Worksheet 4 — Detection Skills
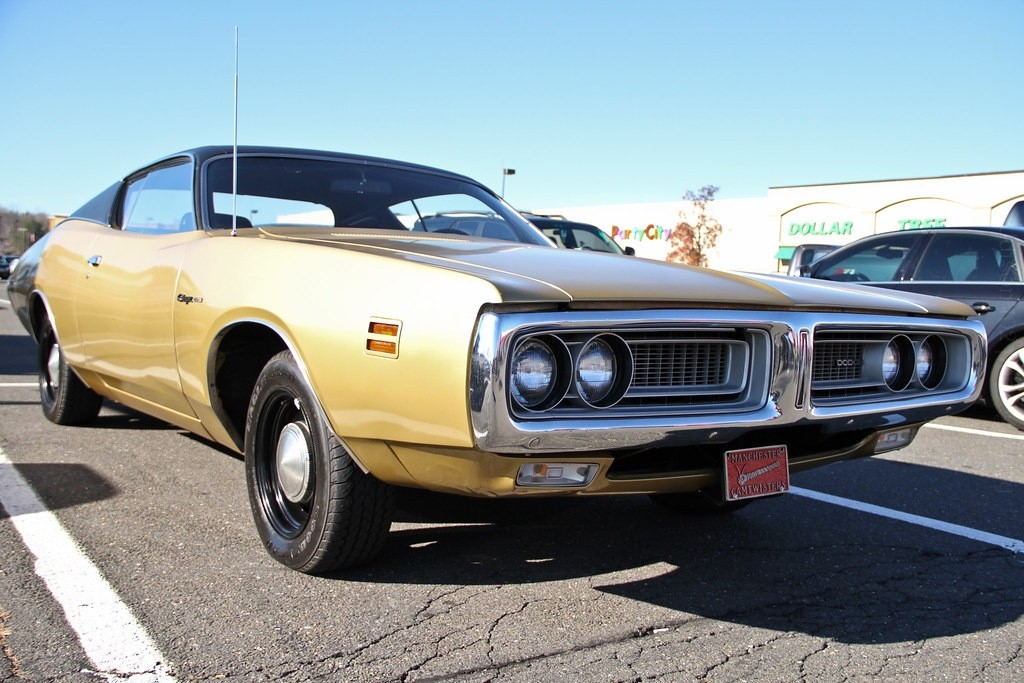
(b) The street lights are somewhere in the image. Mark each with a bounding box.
[502,168,515,200]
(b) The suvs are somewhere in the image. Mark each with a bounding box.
[411,211,636,256]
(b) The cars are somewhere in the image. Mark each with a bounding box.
[7,145,988,578]
[0,261,11,280]
[0,255,20,265]
[800,225,1023,432]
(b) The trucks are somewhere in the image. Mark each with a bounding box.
[788,244,1000,281]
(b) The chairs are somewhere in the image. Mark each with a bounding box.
[178,213,252,232]
[965,250,1002,280]
[916,253,953,281]
